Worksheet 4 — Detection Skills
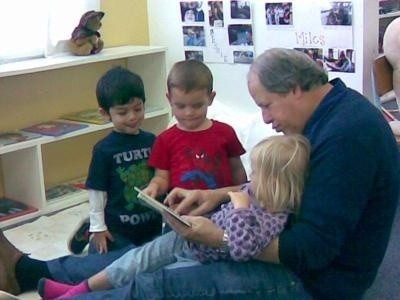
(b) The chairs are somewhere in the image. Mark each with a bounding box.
[370,52,400,143]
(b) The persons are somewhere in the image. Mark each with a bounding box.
[139,59,247,235]
[67,65,159,256]
[0,47,398,299]
[180,1,354,72]
[39,133,312,299]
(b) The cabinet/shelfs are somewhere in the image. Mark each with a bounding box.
[1,41,173,237]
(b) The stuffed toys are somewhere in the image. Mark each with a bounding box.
[69,10,104,55]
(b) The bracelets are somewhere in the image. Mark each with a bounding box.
[217,230,228,253]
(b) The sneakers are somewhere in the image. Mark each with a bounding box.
[0,228,31,295]
[67,216,93,254]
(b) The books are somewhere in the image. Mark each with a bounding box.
[0,107,112,222]
[133,185,191,228]
